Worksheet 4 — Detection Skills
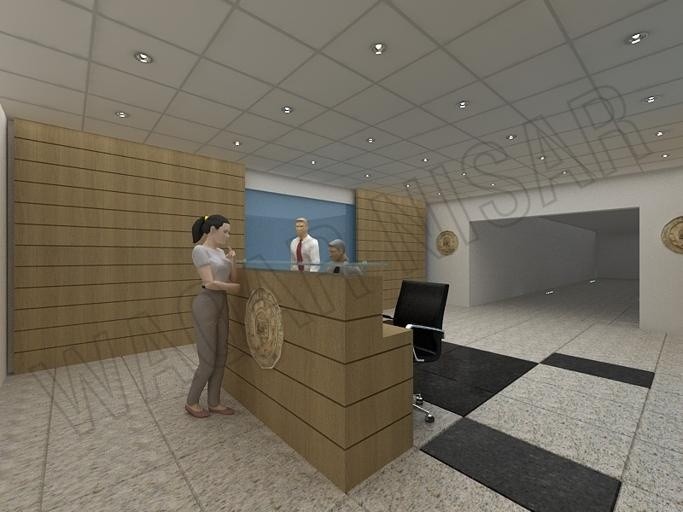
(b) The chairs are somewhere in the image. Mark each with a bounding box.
[382,280,449,421]
